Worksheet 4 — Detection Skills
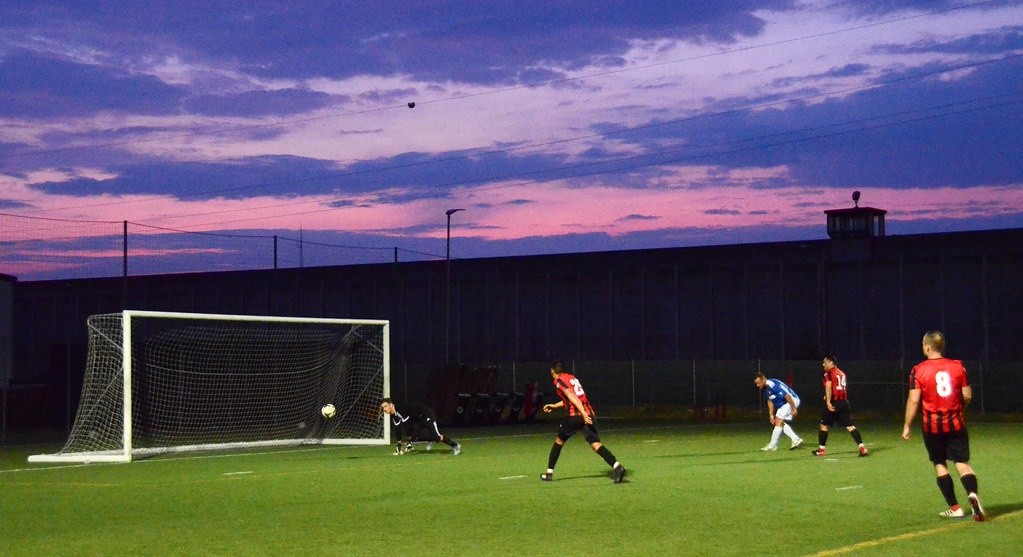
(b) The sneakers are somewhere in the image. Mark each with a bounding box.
[859,447,868,456]
[790,438,803,450]
[967,492,985,522]
[812,448,826,455]
[938,506,964,517]
[540,473,553,481]
[614,466,626,482]
[761,444,777,452]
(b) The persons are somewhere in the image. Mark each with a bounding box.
[811,355,869,457]
[381,397,462,456]
[901,330,986,522]
[754,373,804,452]
[540,360,627,485]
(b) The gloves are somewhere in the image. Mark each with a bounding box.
[405,443,413,452]
[393,444,404,456]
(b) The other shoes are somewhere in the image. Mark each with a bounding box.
[453,443,461,455]
[426,441,433,451]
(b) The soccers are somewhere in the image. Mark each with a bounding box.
[321,404,336,418]
